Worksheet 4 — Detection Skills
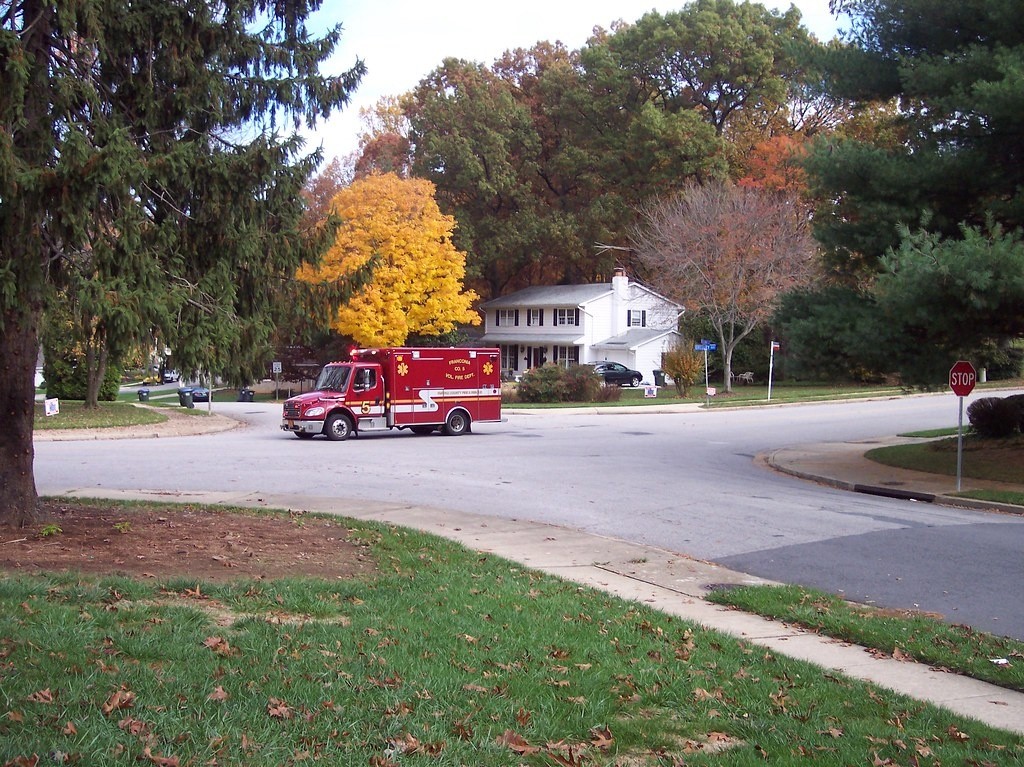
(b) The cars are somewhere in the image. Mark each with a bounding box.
[142,372,167,385]
[162,370,179,383]
[176,386,214,402]
[582,361,643,389]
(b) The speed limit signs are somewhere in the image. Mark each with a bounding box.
[273,362,282,373]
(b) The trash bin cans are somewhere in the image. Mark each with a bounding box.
[177,388,194,407]
[979,367,987,383]
[137,389,150,402]
[238,389,256,402]
[652,369,667,387]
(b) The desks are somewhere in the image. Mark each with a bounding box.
[735,376,742,381]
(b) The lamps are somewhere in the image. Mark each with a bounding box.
[520,345,524,352]
[543,346,547,353]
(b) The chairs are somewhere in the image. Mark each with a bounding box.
[730,372,754,384]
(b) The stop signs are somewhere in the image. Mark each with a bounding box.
[948,361,975,398]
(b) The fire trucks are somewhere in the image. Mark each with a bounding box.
[279,346,507,442]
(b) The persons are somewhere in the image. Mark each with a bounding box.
[355,371,375,391]
